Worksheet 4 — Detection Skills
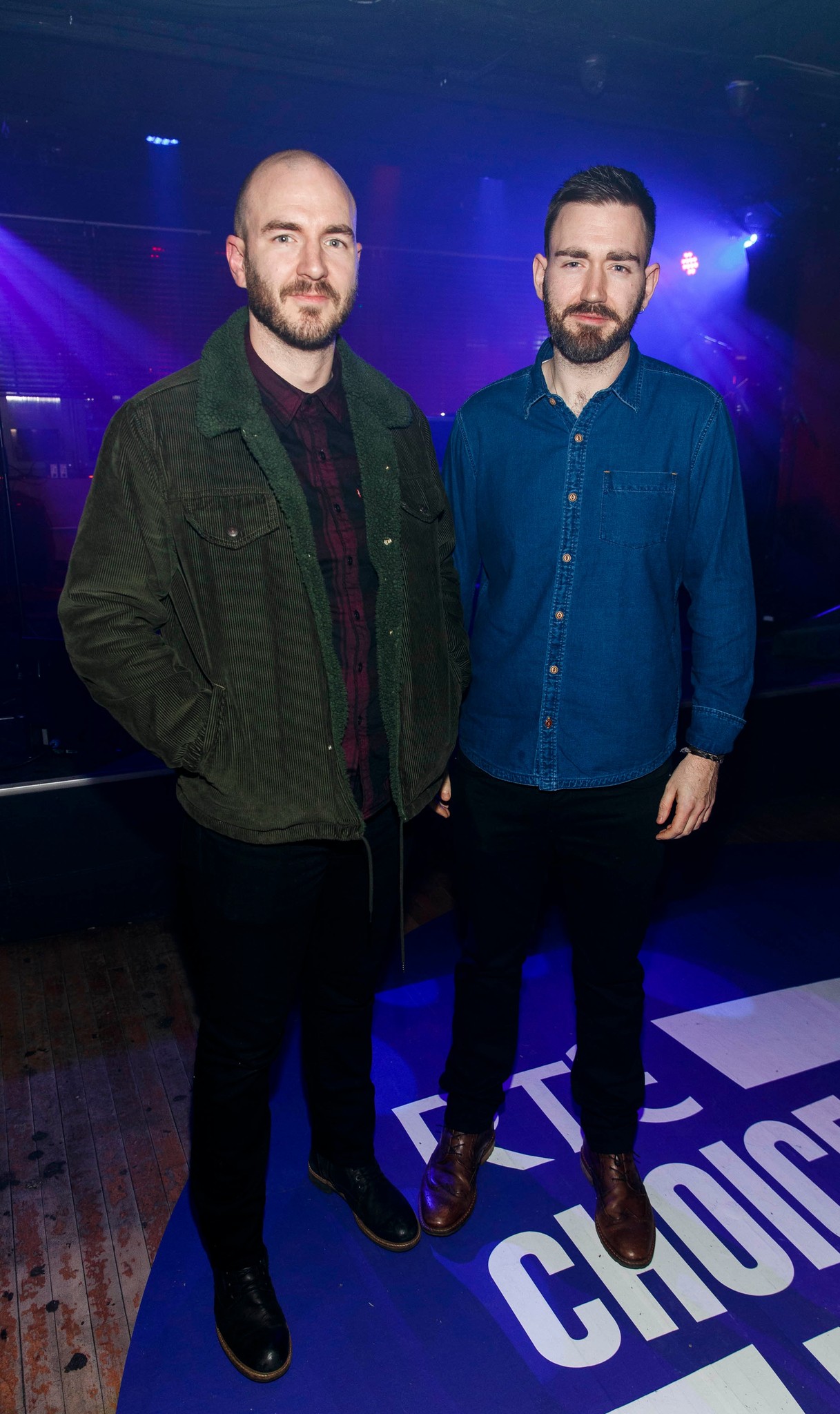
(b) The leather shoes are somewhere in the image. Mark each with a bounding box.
[419,1125,496,1235]
[308,1141,420,1248]
[581,1140,658,1270]
[213,1249,292,1378]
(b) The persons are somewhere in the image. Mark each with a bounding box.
[421,163,755,1269]
[59,149,468,1379]
